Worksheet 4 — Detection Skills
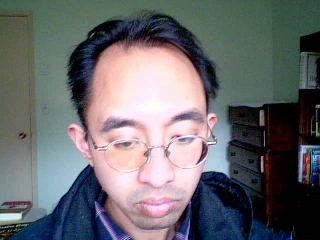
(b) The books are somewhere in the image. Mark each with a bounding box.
[297,145,320,186]
[299,51,320,89]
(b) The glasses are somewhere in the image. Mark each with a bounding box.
[87,124,222,174]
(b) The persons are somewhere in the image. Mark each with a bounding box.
[8,8,294,240]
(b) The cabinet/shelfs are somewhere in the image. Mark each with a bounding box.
[227,102,297,231]
[297,37,320,239]
